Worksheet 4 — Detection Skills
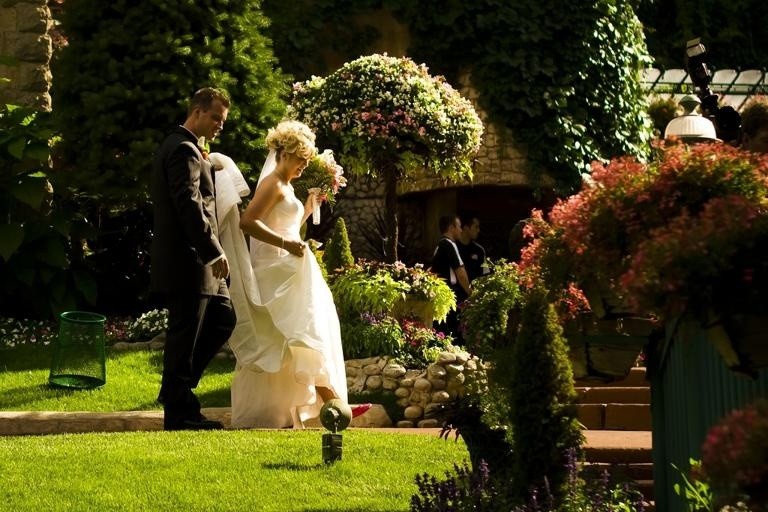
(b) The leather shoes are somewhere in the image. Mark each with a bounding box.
[163,416,225,431]
[351,402,372,419]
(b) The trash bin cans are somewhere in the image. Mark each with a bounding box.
[48,310,108,390]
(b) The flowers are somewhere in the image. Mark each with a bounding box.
[296,150,346,214]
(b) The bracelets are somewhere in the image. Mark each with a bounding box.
[281,236,285,250]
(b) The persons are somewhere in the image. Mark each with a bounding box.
[150,87,230,430]
[739,93,768,154]
[432,210,487,345]
[224,120,371,429]
